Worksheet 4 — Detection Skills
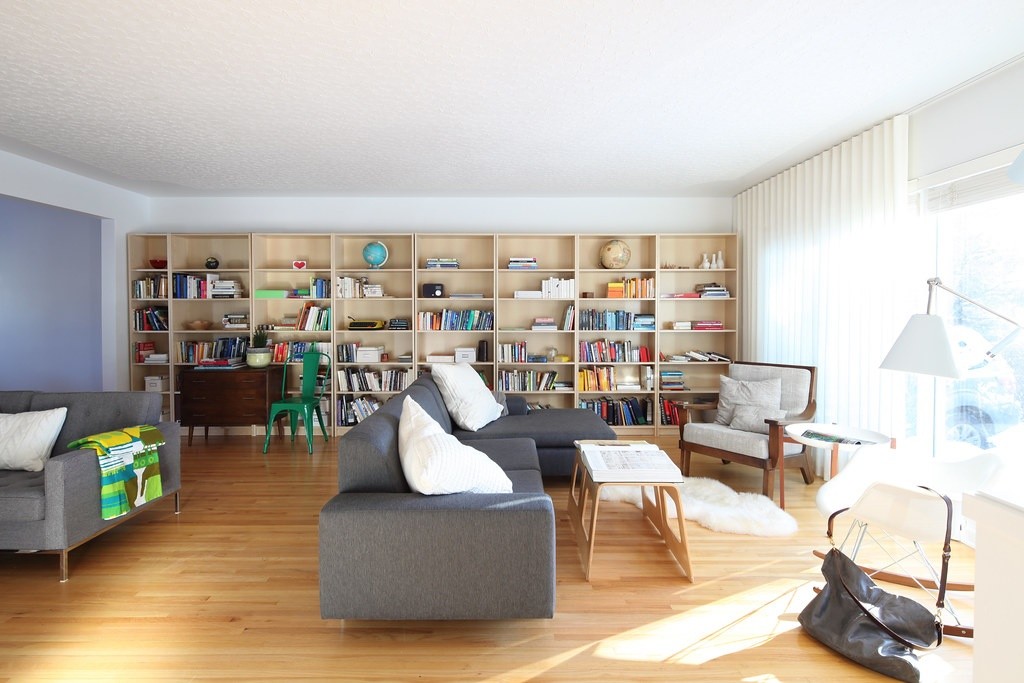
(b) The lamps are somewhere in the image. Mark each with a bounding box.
[877,276,1024,382]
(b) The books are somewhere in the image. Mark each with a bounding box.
[336,276,395,297]
[418,368,431,378]
[337,342,412,426]
[255,272,331,391]
[659,282,731,425]
[132,273,169,363]
[497,257,574,410]
[172,272,250,363]
[418,307,494,330]
[579,275,654,426]
[425,258,460,270]
[477,369,493,391]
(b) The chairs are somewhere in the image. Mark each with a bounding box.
[813,442,975,625]
[262,351,331,456]
[671,360,819,503]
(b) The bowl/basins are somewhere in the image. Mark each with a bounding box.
[187,322,213,330]
[150,259,167,268]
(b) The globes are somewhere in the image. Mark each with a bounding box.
[596,240,633,270]
[363,240,390,271]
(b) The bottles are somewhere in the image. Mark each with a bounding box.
[709,253,717,269]
[699,255,710,269]
[717,250,724,268]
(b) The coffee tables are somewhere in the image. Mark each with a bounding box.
[568,439,695,582]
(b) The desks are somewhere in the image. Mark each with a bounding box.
[785,423,891,480]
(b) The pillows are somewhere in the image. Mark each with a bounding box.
[729,404,789,435]
[713,374,782,426]
[431,364,505,432]
[0,406,68,473]
[397,393,515,496]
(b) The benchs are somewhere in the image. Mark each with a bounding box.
[0,389,183,582]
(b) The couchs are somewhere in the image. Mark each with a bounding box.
[408,372,617,478]
[314,384,555,622]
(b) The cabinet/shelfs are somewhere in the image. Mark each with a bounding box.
[962,469,1024,683]
[577,233,657,437]
[126,233,173,423]
[333,232,415,438]
[251,233,334,436]
[176,365,289,448]
[169,232,253,437]
[495,233,577,408]
[414,232,496,391]
[657,233,739,438]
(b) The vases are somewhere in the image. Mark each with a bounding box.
[710,254,717,269]
[717,250,725,269]
[702,254,710,269]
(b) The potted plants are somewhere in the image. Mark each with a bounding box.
[245,324,273,369]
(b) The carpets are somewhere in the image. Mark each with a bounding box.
[598,475,798,537]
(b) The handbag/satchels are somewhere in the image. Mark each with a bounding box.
[798,484,952,683]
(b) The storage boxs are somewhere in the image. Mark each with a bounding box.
[314,396,328,401]
[292,260,306,269]
[356,345,385,363]
[159,406,171,423]
[305,400,331,414]
[605,282,624,298]
[312,415,330,426]
[381,353,389,362]
[363,284,384,298]
[513,290,542,299]
[426,355,455,363]
[455,347,477,362]
[674,326,692,330]
[583,292,595,298]
[143,374,170,392]
[673,322,692,327]
